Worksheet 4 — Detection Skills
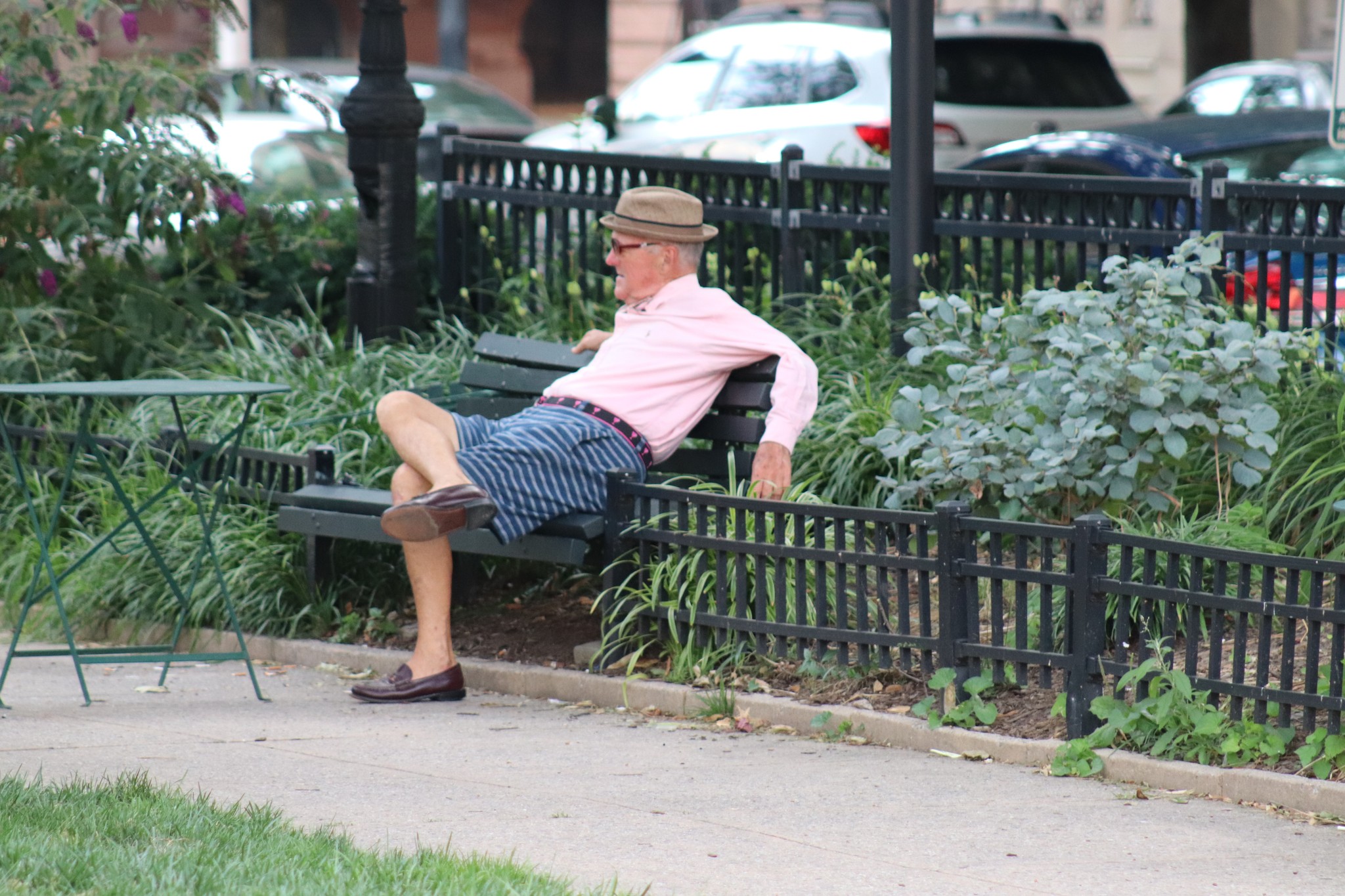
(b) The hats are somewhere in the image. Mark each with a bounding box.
[600,185,718,243]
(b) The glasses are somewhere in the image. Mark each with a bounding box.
[612,238,660,253]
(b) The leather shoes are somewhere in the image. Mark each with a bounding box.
[350,660,468,705]
[380,482,498,544]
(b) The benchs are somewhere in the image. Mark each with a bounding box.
[268,324,785,576]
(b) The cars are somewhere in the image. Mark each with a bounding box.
[941,106,1345,374]
[1158,56,1345,128]
[30,55,537,277]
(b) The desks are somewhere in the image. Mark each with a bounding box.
[0,374,294,709]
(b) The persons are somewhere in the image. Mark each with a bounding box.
[350,185,819,704]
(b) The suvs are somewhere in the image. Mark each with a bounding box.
[494,6,1147,284]
